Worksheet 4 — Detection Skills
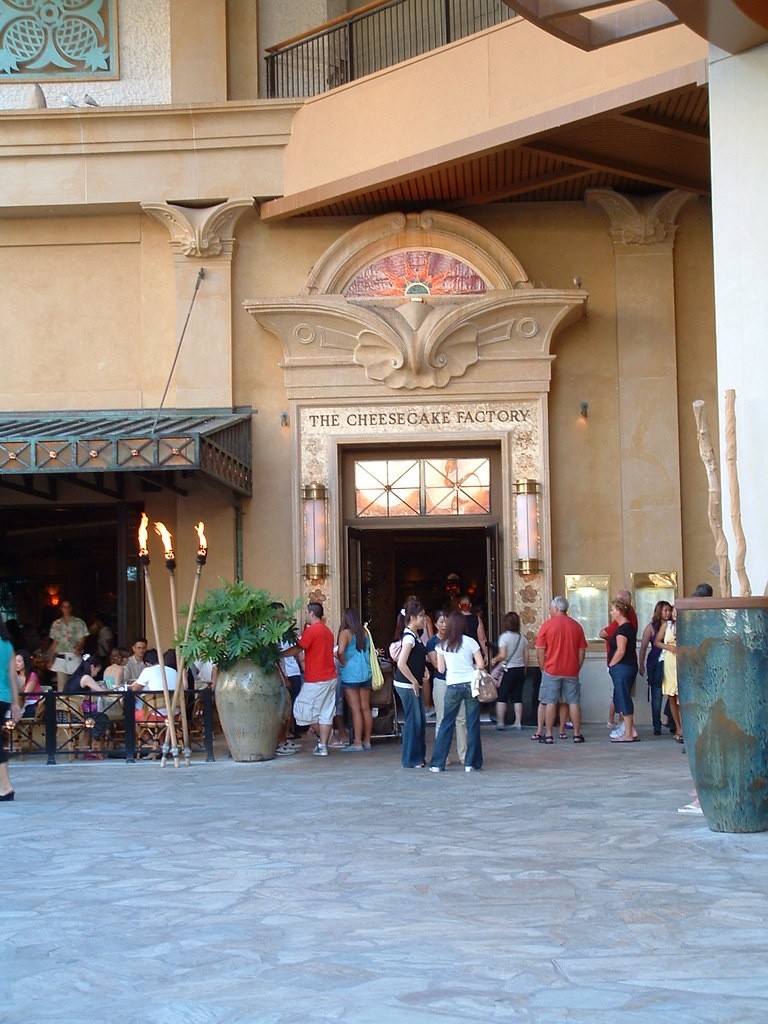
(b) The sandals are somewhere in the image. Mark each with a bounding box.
[559,733,567,739]
[539,735,553,744]
[531,734,545,740]
[573,734,585,742]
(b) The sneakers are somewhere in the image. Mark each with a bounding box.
[275,745,295,755]
[317,729,333,746]
[313,745,328,756]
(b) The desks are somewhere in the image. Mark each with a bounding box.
[97,696,124,721]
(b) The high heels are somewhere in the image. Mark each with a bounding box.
[0,791,15,801]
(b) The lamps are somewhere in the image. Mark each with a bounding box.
[280,411,288,426]
[511,478,543,575]
[300,481,330,581]
[580,402,589,418]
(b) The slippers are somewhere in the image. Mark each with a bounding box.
[612,737,640,743]
[677,804,703,813]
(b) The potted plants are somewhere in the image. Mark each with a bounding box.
[170,577,306,762]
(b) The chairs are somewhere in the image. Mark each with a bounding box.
[105,693,204,761]
[1,695,104,763]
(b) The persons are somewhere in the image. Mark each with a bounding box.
[427,611,468,765]
[258,602,350,755]
[46,600,91,691]
[394,593,489,717]
[429,610,485,773]
[392,601,430,769]
[85,612,218,759]
[279,602,338,756]
[5,618,58,730]
[491,612,529,730]
[531,583,713,744]
[0,611,23,802]
[63,654,111,761]
[337,607,385,753]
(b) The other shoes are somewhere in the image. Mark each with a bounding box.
[464,766,474,773]
[496,723,506,730]
[143,753,162,760]
[429,766,440,773]
[610,722,625,738]
[328,736,349,748]
[362,740,371,751]
[672,733,683,743]
[402,762,425,768]
[507,724,522,730]
[339,744,364,752]
[607,721,617,729]
[284,742,304,752]
[83,753,107,760]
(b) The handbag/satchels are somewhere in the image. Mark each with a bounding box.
[82,700,97,712]
[365,627,385,691]
[491,662,505,688]
[478,667,498,703]
